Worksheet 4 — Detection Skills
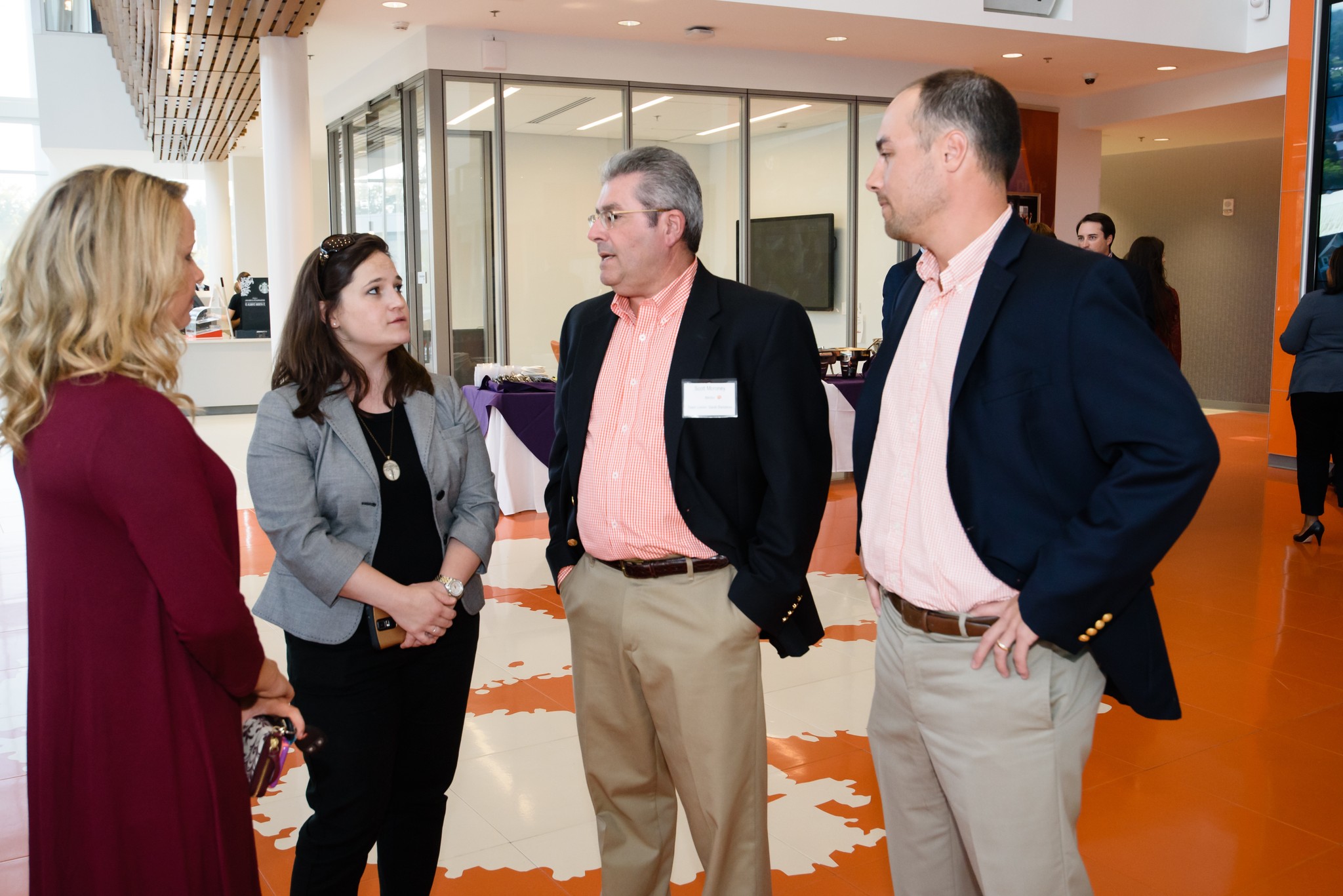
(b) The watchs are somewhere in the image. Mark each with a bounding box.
[434,574,464,599]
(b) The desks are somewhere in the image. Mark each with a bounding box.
[461,385,555,515]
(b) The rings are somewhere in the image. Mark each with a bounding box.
[431,626,438,635]
[996,639,1011,652]
[427,633,434,638]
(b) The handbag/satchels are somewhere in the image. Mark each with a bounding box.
[242,714,298,799]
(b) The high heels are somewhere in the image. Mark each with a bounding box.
[1293,520,1325,547]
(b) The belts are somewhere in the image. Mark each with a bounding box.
[595,553,731,579]
[886,591,999,638]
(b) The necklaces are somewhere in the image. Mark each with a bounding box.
[350,388,401,481]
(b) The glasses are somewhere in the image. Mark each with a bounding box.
[588,207,671,231]
[318,232,361,288]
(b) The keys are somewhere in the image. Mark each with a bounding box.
[272,717,295,741]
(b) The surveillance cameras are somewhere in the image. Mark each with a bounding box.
[1080,72,1099,85]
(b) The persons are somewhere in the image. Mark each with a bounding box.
[1278,244,1343,547]
[1076,212,1120,259]
[245,224,497,896]
[228,271,252,337]
[1122,236,1183,369]
[195,282,209,291]
[1027,222,1058,239]
[0,163,309,896]
[848,66,1227,895]
[545,147,837,896]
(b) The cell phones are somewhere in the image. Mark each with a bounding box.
[365,603,407,651]
